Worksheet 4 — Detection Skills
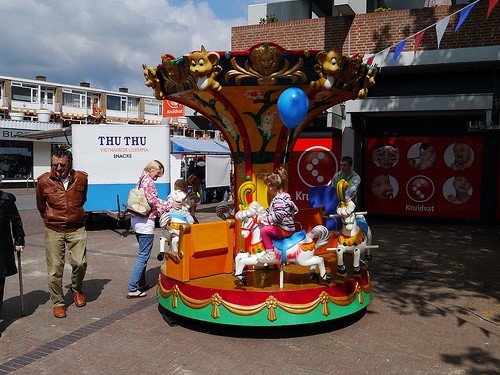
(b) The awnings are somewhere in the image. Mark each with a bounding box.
[15,126,72,140]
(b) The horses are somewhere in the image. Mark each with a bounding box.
[235,200,330,284]
[335,197,373,275]
[157,208,195,262]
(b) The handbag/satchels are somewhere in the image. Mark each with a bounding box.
[127,175,153,218]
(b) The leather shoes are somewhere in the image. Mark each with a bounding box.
[70,284,87,307]
[54,306,66,318]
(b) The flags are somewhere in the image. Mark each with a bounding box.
[162,100,185,117]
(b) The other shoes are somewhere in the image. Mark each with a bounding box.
[257,253,277,263]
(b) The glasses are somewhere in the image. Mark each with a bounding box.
[340,163,349,167]
[54,163,66,167]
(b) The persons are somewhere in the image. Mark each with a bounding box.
[181,157,236,203]
[0,171,25,316]
[126,159,181,298]
[330,156,362,211]
[159,177,195,227]
[36,148,88,318]
[257,167,299,263]
[372,142,473,203]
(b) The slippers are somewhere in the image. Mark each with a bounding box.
[127,291,147,299]
[137,284,153,290]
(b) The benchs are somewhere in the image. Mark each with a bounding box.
[164,217,236,283]
[296,205,339,255]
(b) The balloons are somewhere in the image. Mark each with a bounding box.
[278,87,309,130]
